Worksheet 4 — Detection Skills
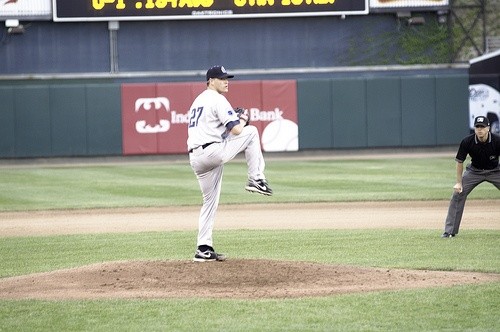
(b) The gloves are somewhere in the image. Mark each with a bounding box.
[234,107,250,126]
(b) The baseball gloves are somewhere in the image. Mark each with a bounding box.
[236,106,250,126]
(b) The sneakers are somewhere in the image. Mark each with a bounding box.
[194,250,227,262]
[246,178,272,195]
[440,232,455,238]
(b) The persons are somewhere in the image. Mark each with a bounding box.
[441,116,500,238]
[186,65,273,261]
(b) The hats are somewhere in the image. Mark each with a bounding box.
[474,116,489,127]
[206,66,235,81]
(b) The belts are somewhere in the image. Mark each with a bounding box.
[189,143,212,153]
